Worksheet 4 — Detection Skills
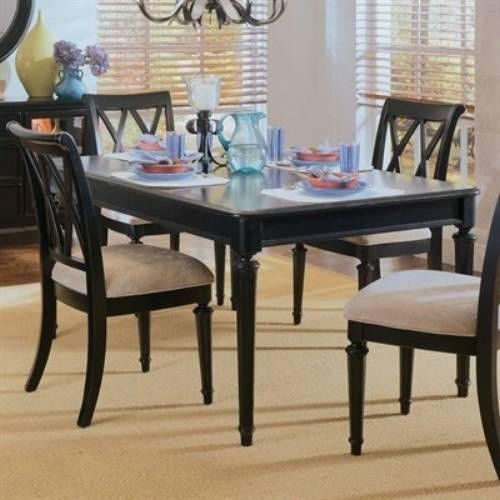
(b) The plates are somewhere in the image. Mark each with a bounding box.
[135,135,193,176]
[292,150,367,194]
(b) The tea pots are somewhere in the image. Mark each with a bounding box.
[219,109,268,172]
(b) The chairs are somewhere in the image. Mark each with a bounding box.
[290,95,465,325]
[84,92,183,254]
[340,189,499,484]
[7,121,216,430]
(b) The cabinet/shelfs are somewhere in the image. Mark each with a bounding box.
[0,98,100,232]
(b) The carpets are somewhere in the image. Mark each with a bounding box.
[2,257,500,500]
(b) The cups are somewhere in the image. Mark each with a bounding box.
[266,127,284,158]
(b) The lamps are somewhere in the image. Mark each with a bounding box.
[138,0,288,28]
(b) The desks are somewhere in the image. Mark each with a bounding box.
[29,146,481,448]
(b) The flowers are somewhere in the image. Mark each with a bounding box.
[54,42,109,77]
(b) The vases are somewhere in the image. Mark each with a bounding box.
[53,68,86,98]
[16,10,57,98]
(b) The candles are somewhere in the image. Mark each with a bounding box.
[181,75,227,111]
